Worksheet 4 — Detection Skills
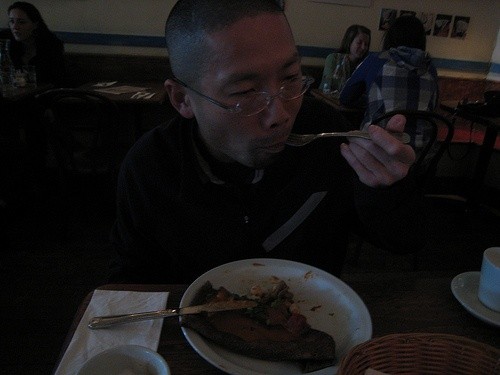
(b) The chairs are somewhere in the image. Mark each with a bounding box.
[0,86,454,270]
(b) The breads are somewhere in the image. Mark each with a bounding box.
[177,280,336,361]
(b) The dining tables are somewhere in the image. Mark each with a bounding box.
[50,276,500,375]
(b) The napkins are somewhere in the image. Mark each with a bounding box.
[54,289,169,375]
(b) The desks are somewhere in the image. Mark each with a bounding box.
[421,100,500,220]
[311,89,358,113]
[74,81,171,142]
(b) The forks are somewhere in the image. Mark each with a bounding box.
[286,130,411,146]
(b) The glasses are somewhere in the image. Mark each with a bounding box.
[171,75,315,116]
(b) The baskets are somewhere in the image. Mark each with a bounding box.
[336,332,500,375]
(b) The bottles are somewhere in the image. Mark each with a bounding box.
[0,39,15,93]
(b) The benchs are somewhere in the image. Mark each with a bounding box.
[78,53,500,161]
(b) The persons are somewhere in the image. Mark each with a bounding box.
[316,24,371,98]
[338,16,439,159]
[0,2,66,164]
[111,0,436,285]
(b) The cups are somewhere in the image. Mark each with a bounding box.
[478,247,500,312]
[23,64,36,83]
[78,345,172,375]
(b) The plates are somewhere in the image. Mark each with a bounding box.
[450,271,500,329]
[179,258,373,375]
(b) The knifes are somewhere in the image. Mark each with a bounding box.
[87,300,259,329]
